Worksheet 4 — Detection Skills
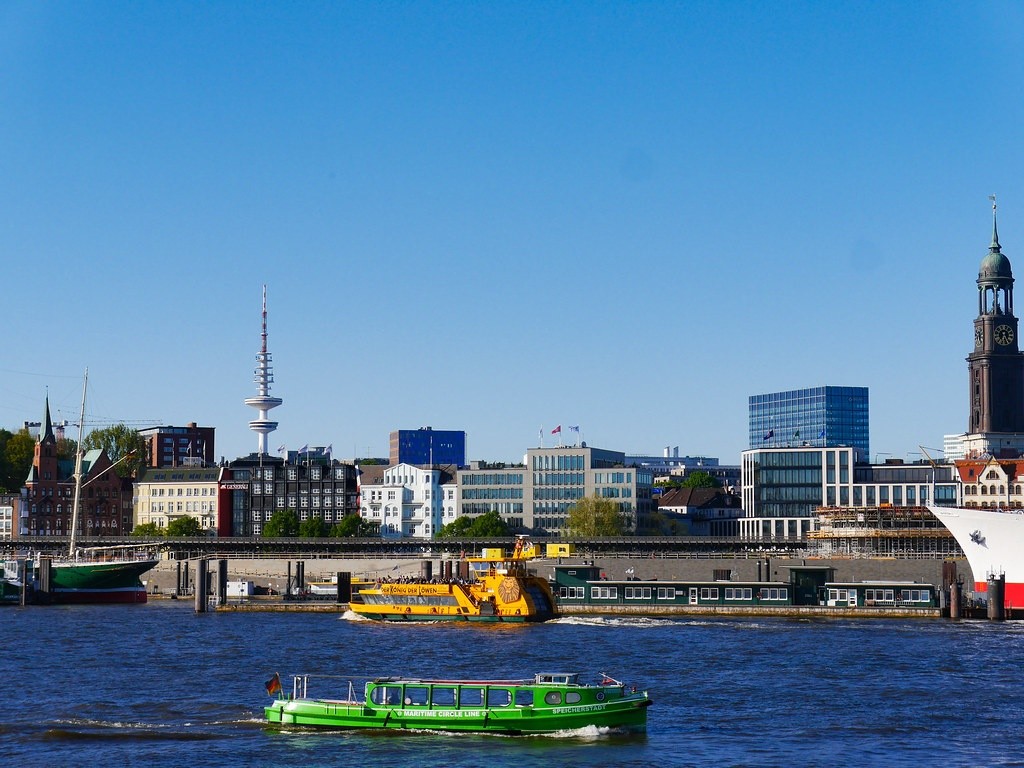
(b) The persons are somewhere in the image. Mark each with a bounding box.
[516,538,533,551]
[377,575,480,589]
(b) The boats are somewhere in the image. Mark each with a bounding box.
[348,535,559,624]
[262,671,654,736]
[925,504,1024,609]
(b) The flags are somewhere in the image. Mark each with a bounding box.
[625,566,634,574]
[539,428,543,437]
[277,445,285,453]
[795,429,799,436]
[551,425,561,434]
[186,443,192,453]
[819,432,825,438]
[392,564,398,570]
[568,425,579,433]
[324,444,332,455]
[764,429,774,440]
[264,674,281,696]
[298,444,308,454]
[203,443,206,455]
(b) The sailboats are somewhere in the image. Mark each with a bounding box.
[0,366,161,605]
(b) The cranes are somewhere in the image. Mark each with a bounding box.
[23,409,164,453]
